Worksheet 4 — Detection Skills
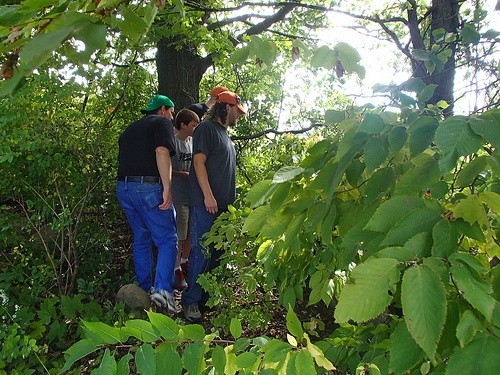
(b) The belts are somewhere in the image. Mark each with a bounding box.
[117,175,161,183]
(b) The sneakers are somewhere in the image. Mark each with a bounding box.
[202,297,227,311]
[183,305,202,323]
[180,257,191,276]
[146,286,182,302]
[174,266,188,290]
[149,288,177,314]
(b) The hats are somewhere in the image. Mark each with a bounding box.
[218,91,246,114]
[211,86,229,97]
[140,94,175,114]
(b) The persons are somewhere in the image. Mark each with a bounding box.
[116,95,178,314]
[153,86,229,291]
[181,90,248,322]
